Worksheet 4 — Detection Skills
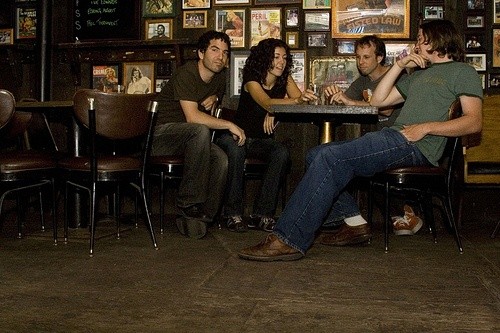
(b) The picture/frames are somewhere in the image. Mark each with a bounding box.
[0,0,39,45]
[89,0,500,99]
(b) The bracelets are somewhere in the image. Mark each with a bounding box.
[396,61,406,69]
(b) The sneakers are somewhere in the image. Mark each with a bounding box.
[402,205,423,233]
[249,214,276,232]
[227,216,249,232]
[238,232,303,261]
[393,217,414,235]
[320,222,373,247]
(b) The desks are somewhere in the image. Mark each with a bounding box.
[265,104,378,145]
[15,101,79,157]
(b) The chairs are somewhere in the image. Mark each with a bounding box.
[0,89,500,255]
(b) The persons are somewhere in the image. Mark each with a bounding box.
[225,12,243,36]
[0,31,10,43]
[324,34,423,235]
[224,37,320,231]
[237,19,483,262]
[186,14,202,25]
[127,66,151,94]
[150,24,169,40]
[103,68,118,93]
[149,30,245,240]
[466,36,481,48]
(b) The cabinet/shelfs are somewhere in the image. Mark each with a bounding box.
[58,38,190,93]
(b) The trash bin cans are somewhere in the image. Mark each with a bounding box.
[67,0,142,43]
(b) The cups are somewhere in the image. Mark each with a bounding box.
[310,84,323,105]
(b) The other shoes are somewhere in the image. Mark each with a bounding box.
[174,203,213,222]
[176,216,207,240]
